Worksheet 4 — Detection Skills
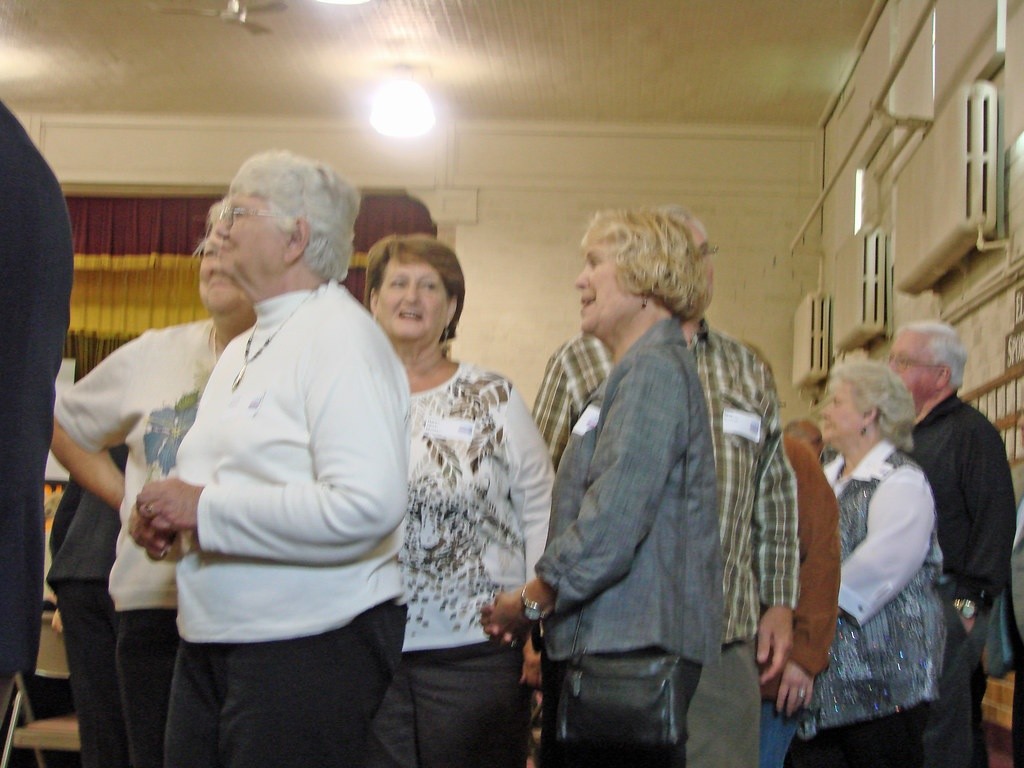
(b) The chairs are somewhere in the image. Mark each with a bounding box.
[0,612,83,768]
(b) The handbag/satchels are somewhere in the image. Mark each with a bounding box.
[554,653,688,747]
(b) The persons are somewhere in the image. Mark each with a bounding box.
[534,205,801,768]
[131,150,413,768]
[0,102,74,677]
[886,321,1017,768]
[479,204,725,768]
[363,235,555,768]
[760,419,842,768]
[790,356,947,768]
[48,200,258,768]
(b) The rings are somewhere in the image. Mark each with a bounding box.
[511,639,517,647]
[144,502,153,512]
[798,689,805,698]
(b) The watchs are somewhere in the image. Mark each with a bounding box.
[952,599,977,620]
[521,585,546,621]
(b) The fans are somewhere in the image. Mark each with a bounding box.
[157,0,285,35]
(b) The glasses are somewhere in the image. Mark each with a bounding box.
[887,351,950,372]
[218,202,297,229]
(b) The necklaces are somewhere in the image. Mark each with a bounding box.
[231,288,316,390]
